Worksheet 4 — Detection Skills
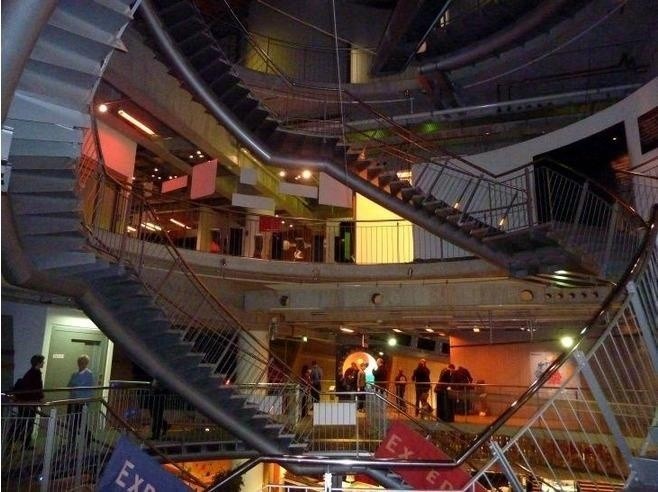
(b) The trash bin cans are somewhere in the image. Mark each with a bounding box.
[241,214,274,259]
[282,384,301,427]
[350,45,376,85]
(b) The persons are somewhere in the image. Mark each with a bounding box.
[62,354,95,450]
[335,356,473,423]
[297,365,313,417]
[3,354,47,457]
[310,360,323,403]
[146,378,172,441]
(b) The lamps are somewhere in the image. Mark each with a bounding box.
[169,218,192,231]
[117,109,156,136]
